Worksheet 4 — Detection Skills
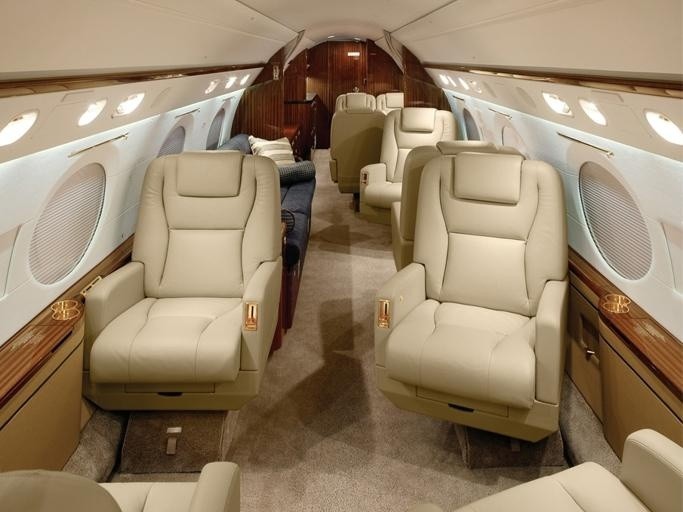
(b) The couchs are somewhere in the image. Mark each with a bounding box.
[216,133,316,357]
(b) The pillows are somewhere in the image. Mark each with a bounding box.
[248,135,314,188]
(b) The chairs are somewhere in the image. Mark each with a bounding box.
[413,428,681,511]
[83,155,282,413]
[331,94,404,214]
[359,107,457,227]
[373,150,571,445]
[1,460,240,510]
[390,140,526,273]
